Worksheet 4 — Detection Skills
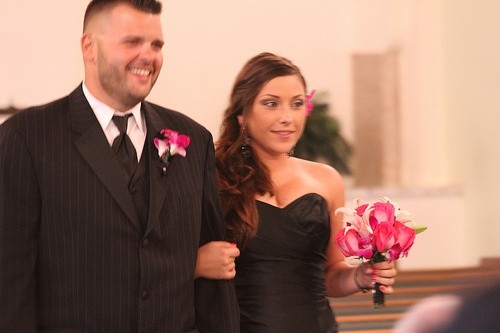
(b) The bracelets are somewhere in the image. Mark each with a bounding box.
[355,266,371,293]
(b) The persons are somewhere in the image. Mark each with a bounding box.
[0,0,241,333]
[195,53,397,333]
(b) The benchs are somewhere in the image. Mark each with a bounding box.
[329,259,500,333]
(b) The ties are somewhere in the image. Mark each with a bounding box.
[111,113,138,176]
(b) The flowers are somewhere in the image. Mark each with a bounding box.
[153,128,191,168]
[334,197,427,308]
